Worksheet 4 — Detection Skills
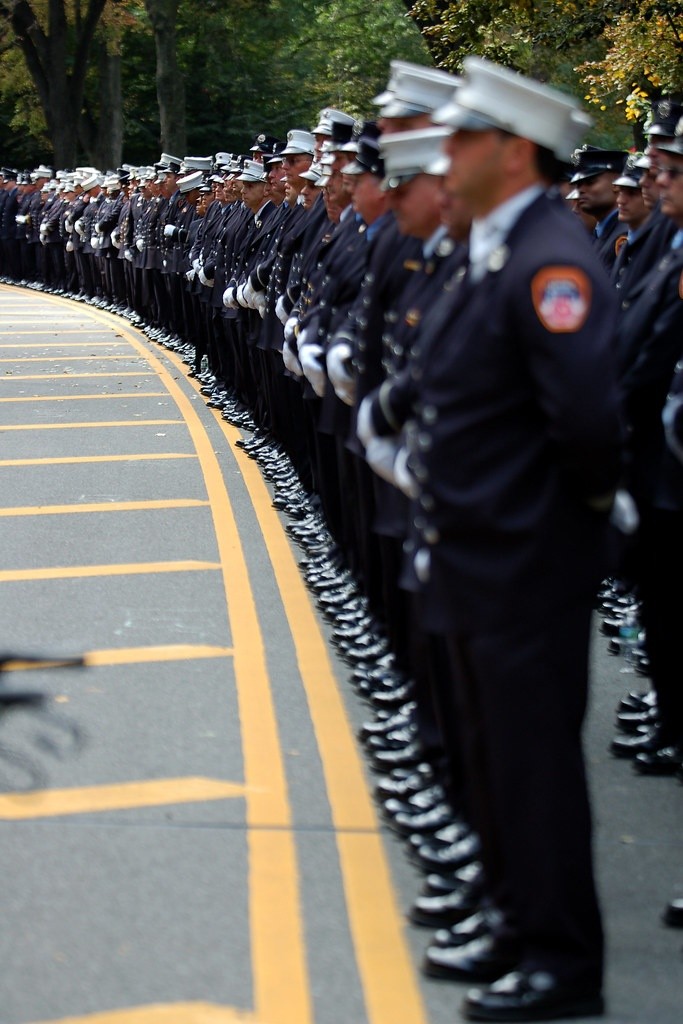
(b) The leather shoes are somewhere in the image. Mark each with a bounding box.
[594,576,683,928]
[269,474,607,1023]
[2,273,292,473]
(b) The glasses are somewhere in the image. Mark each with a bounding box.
[648,164,682,181]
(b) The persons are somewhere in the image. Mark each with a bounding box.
[2,59,682,1023]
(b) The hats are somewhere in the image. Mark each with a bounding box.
[0,54,682,199]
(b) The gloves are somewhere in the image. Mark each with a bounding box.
[11,212,683,534]
